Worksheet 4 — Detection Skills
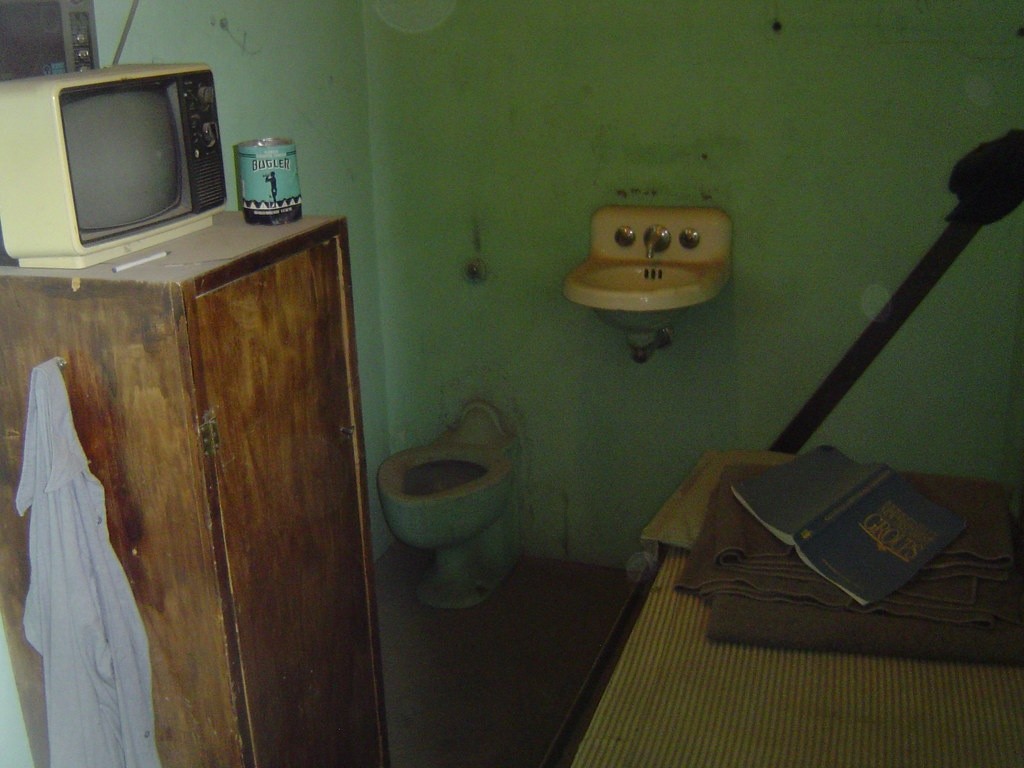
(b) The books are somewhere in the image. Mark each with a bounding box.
[731,444,969,607]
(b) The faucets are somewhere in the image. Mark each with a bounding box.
[643,233,661,260]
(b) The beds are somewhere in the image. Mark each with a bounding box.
[534,446,1024,768]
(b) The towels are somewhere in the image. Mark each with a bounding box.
[674,458,1024,663]
[15,353,162,768]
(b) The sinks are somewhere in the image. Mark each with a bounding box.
[560,256,719,330]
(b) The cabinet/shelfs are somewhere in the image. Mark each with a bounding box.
[0,207,390,768]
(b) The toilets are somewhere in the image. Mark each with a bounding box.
[377,394,523,608]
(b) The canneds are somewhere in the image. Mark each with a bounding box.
[238,137,303,226]
